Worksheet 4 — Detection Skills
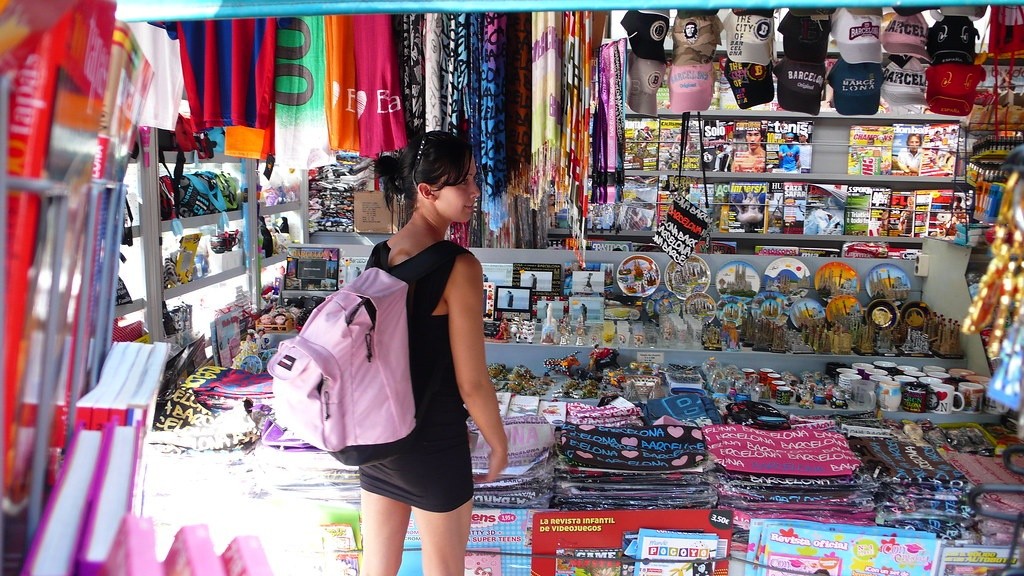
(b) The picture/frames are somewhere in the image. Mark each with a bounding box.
[495,285,533,322]
[512,262,562,296]
[563,260,614,297]
[483,282,494,321]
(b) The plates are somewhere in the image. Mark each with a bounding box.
[750,291,790,326]
[865,298,899,332]
[683,292,716,325]
[714,260,760,304]
[813,262,861,304]
[664,253,712,301]
[789,297,826,330]
[763,257,811,303]
[644,288,681,327]
[826,294,863,332]
[715,295,748,328]
[617,254,662,298]
[899,300,933,332]
[864,262,911,306]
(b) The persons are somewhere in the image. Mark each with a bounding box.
[633,125,733,172]
[897,135,922,178]
[776,133,801,176]
[796,130,812,174]
[731,125,765,175]
[359,131,510,576]
[929,126,959,161]
[862,139,882,158]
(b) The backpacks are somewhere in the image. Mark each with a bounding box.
[267,239,475,466]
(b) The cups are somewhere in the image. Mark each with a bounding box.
[741,364,796,405]
[824,361,1010,415]
[601,314,703,348]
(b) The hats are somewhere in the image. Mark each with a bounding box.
[724,58,775,110]
[832,8,884,64]
[772,54,827,117]
[827,57,883,116]
[621,8,670,63]
[777,11,832,65]
[928,9,981,66]
[881,12,931,63]
[880,59,930,110]
[672,13,722,66]
[669,64,716,114]
[624,51,666,119]
[723,11,776,67]
[924,64,986,117]
